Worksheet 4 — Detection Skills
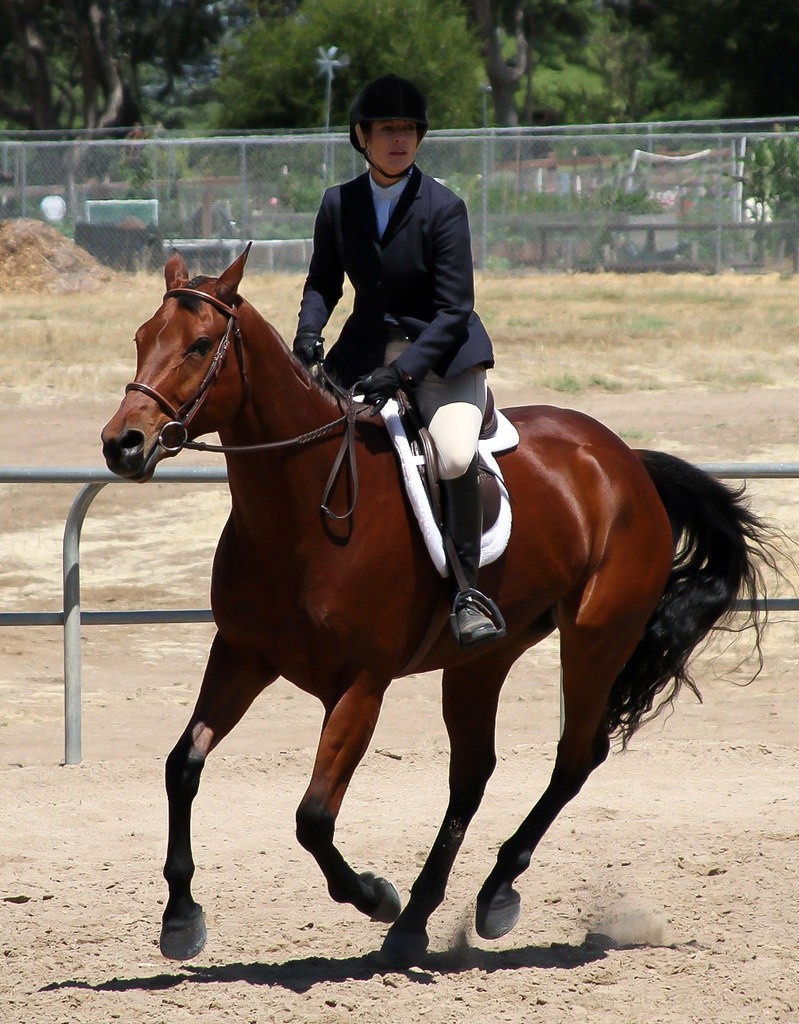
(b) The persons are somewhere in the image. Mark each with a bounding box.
[189,187,240,278]
[293,71,499,645]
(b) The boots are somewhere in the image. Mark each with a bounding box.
[439,452,497,643]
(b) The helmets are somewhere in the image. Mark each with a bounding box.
[350,73,429,156]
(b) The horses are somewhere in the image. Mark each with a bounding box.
[100,240,799,967]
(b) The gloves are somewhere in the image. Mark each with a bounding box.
[353,364,405,417]
[293,332,325,368]
